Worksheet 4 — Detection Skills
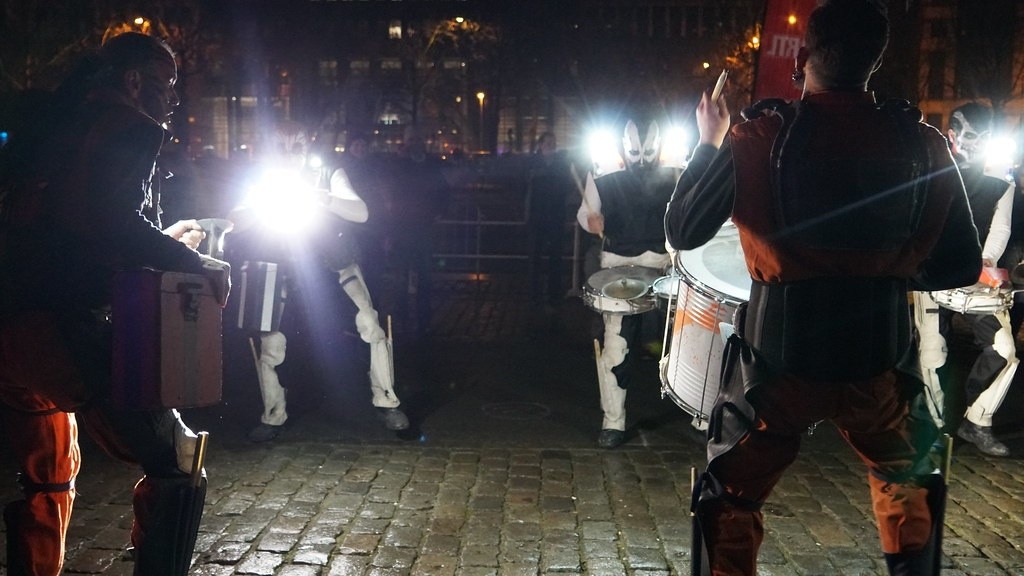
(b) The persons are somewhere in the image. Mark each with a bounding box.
[664,0,986,576]
[909,103,1019,456]
[337,133,396,267]
[0,27,234,576]
[216,120,414,441]
[527,132,570,247]
[575,115,692,450]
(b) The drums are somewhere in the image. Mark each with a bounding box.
[658,218,754,434]
[928,265,1014,315]
[578,264,665,315]
[652,276,678,318]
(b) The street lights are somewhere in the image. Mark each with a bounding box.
[477,91,486,151]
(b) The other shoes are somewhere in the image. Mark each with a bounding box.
[373,400,410,431]
[597,429,625,449]
[247,416,291,442]
[929,419,961,454]
[956,416,1011,457]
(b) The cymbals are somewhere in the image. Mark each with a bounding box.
[601,277,649,301]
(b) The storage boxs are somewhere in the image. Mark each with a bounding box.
[112,268,224,412]
